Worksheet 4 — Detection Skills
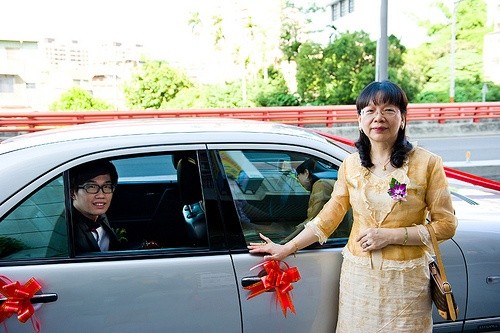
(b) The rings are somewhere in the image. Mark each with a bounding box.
[265,243,266,245]
[366,242,369,246]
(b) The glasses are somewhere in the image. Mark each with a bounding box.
[77,184,117,194]
[361,106,401,118]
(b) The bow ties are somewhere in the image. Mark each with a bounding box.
[89,217,106,231]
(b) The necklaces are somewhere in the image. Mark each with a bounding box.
[370,152,390,170]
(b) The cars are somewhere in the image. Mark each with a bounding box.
[0,113,499,333]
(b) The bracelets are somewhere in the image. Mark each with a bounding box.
[289,240,298,251]
[402,227,408,246]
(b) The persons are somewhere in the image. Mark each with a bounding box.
[248,81,456,333]
[71,161,123,255]
[279,158,353,246]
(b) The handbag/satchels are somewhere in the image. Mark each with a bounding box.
[426,224,459,321]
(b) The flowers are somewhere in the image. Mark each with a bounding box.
[387,178,408,203]
